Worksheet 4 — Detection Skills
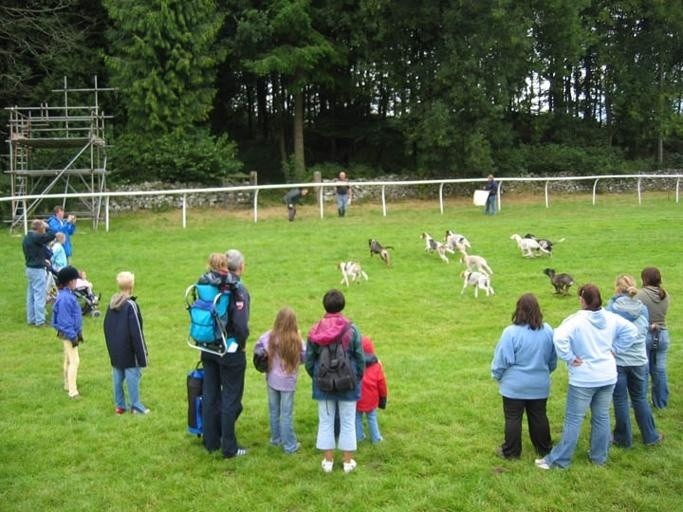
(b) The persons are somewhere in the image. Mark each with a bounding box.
[76,271,99,304]
[608,274,665,448]
[50,232,67,272]
[331,172,352,217]
[103,270,149,415]
[46,205,76,266]
[483,175,498,216]
[42,221,56,304]
[305,289,364,473]
[200,249,251,457]
[356,336,387,445]
[283,188,309,222]
[254,308,306,453]
[491,294,558,461]
[628,267,670,409]
[534,284,638,470]
[199,253,244,310]
[22,220,57,328]
[51,266,83,401]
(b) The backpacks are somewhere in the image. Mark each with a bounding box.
[317,321,357,393]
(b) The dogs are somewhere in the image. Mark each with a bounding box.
[364,237,398,267]
[441,229,471,257]
[457,268,496,301]
[457,254,496,279]
[417,229,455,265]
[523,233,567,261]
[507,233,551,259]
[334,259,370,290]
[541,267,580,299]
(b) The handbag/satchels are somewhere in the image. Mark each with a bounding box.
[253,342,270,372]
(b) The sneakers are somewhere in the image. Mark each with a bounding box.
[236,449,246,457]
[70,395,83,401]
[372,436,382,444]
[497,447,518,460]
[357,433,367,443]
[612,434,632,448]
[321,458,334,472]
[535,458,552,470]
[270,438,280,446]
[114,406,125,416]
[587,450,606,468]
[286,443,301,454]
[131,408,151,414]
[37,322,50,327]
[646,433,663,445]
[343,459,357,472]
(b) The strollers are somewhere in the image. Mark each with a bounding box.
[46,263,102,319]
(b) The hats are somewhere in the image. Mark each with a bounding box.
[58,265,82,281]
